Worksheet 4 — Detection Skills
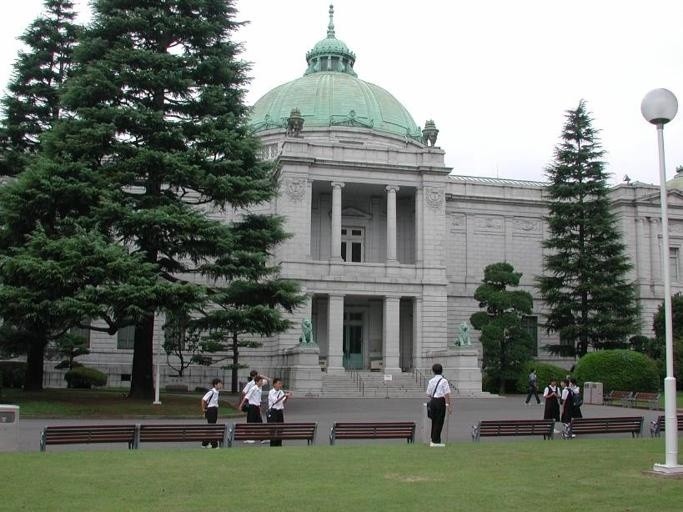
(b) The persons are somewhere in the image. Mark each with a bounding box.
[200,378,222,449]
[568,378,583,419]
[237,374,270,444]
[522,366,545,405]
[265,377,293,447]
[541,375,561,437]
[558,378,578,438]
[424,362,453,448]
[239,369,271,401]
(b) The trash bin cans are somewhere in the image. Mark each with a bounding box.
[582,381,603,404]
[0,404,20,451]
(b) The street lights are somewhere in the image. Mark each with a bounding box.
[640,88,679,466]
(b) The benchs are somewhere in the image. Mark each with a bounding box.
[38,419,319,453]
[328,422,417,444]
[604,389,661,411]
[468,417,556,440]
[651,412,683,439]
[563,416,646,440]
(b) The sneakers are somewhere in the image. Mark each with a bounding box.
[430,441,445,447]
[244,439,256,443]
[562,433,576,438]
[261,439,270,443]
[553,428,560,433]
[201,444,219,448]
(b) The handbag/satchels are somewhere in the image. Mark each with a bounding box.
[570,390,583,407]
[427,401,432,417]
[242,402,250,412]
